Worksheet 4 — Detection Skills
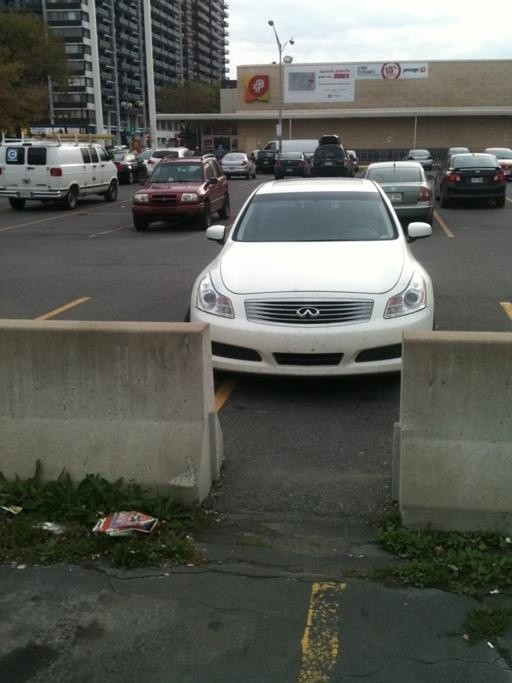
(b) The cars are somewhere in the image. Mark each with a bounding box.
[402,149,433,171]
[434,153,506,207]
[366,160,434,221]
[274,152,310,179]
[346,150,360,171]
[485,147,512,178]
[185,178,434,376]
[219,153,256,179]
[114,152,144,183]
[443,147,471,164]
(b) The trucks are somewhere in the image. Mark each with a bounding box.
[262,139,319,153]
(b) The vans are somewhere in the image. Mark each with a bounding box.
[0,131,119,208]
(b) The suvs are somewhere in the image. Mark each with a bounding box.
[311,135,354,176]
[132,153,230,229]
[146,147,192,177]
[255,150,278,173]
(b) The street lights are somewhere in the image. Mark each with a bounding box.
[268,19,295,138]
